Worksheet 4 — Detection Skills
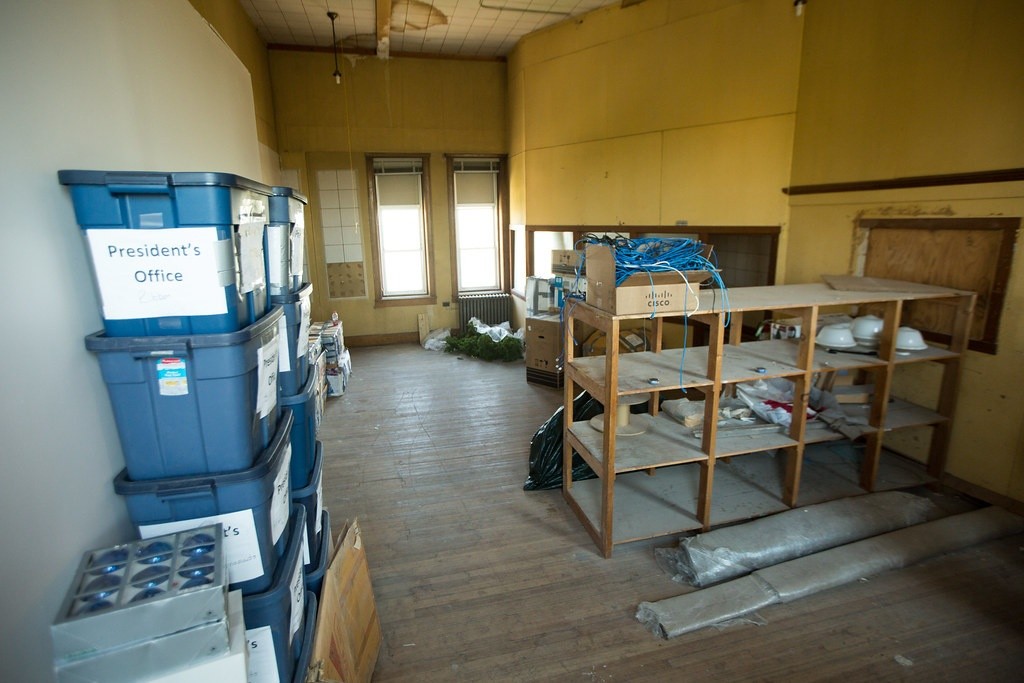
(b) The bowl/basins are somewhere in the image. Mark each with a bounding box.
[816,327,856,349]
[895,327,929,355]
[852,315,885,347]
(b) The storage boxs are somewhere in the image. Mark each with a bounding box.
[524,310,598,388]
[585,243,726,314]
[551,249,588,274]
[57,168,351,683]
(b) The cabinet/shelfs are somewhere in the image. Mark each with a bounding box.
[562,276,978,558]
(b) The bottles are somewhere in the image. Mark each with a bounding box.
[332,311,338,326]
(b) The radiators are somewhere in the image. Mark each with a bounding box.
[456,293,513,339]
